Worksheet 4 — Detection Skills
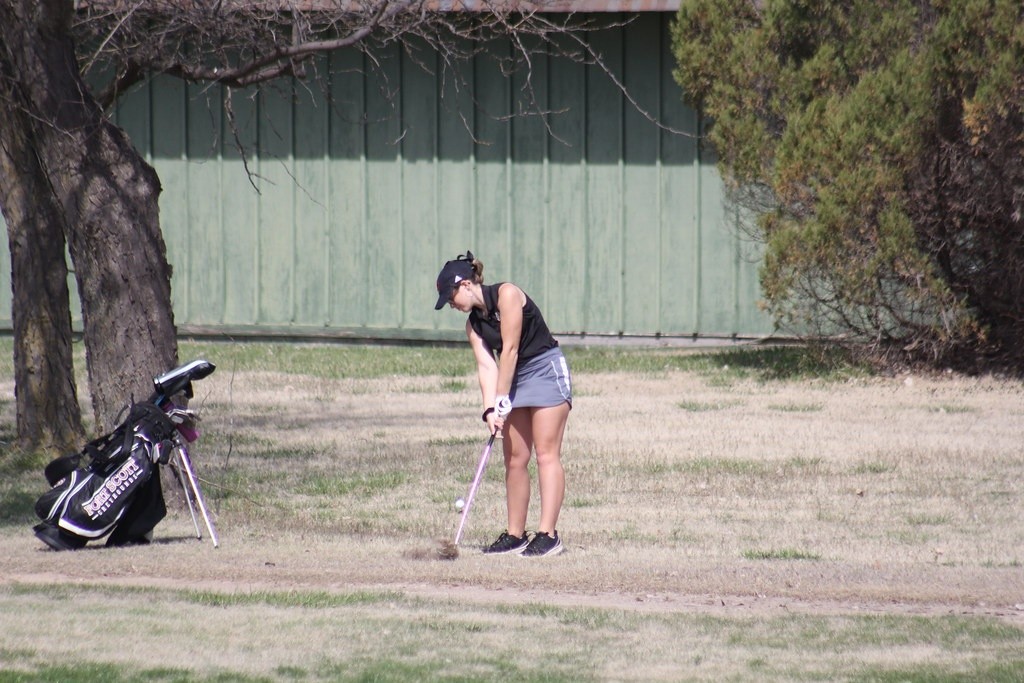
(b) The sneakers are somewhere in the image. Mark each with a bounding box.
[482,530,528,557]
[517,530,565,559]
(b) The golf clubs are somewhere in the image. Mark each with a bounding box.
[437,396,505,558]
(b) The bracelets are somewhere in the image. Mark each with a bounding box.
[482,406,494,422]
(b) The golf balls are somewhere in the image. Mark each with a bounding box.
[455,499,466,512]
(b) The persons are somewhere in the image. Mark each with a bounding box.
[435,249,574,560]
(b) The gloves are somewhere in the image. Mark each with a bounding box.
[493,396,512,424]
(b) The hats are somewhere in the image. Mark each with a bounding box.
[434,259,476,312]
